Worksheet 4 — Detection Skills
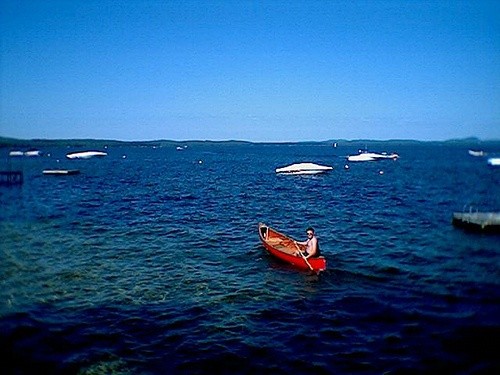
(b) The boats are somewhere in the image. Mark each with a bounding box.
[276,162,334,175]
[66,150,108,159]
[258,221,327,272]
[348,144,400,162]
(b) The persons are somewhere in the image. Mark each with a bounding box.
[294,227,319,259]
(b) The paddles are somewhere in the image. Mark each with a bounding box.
[294,241,318,275]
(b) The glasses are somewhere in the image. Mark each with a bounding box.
[306,232,313,236]
